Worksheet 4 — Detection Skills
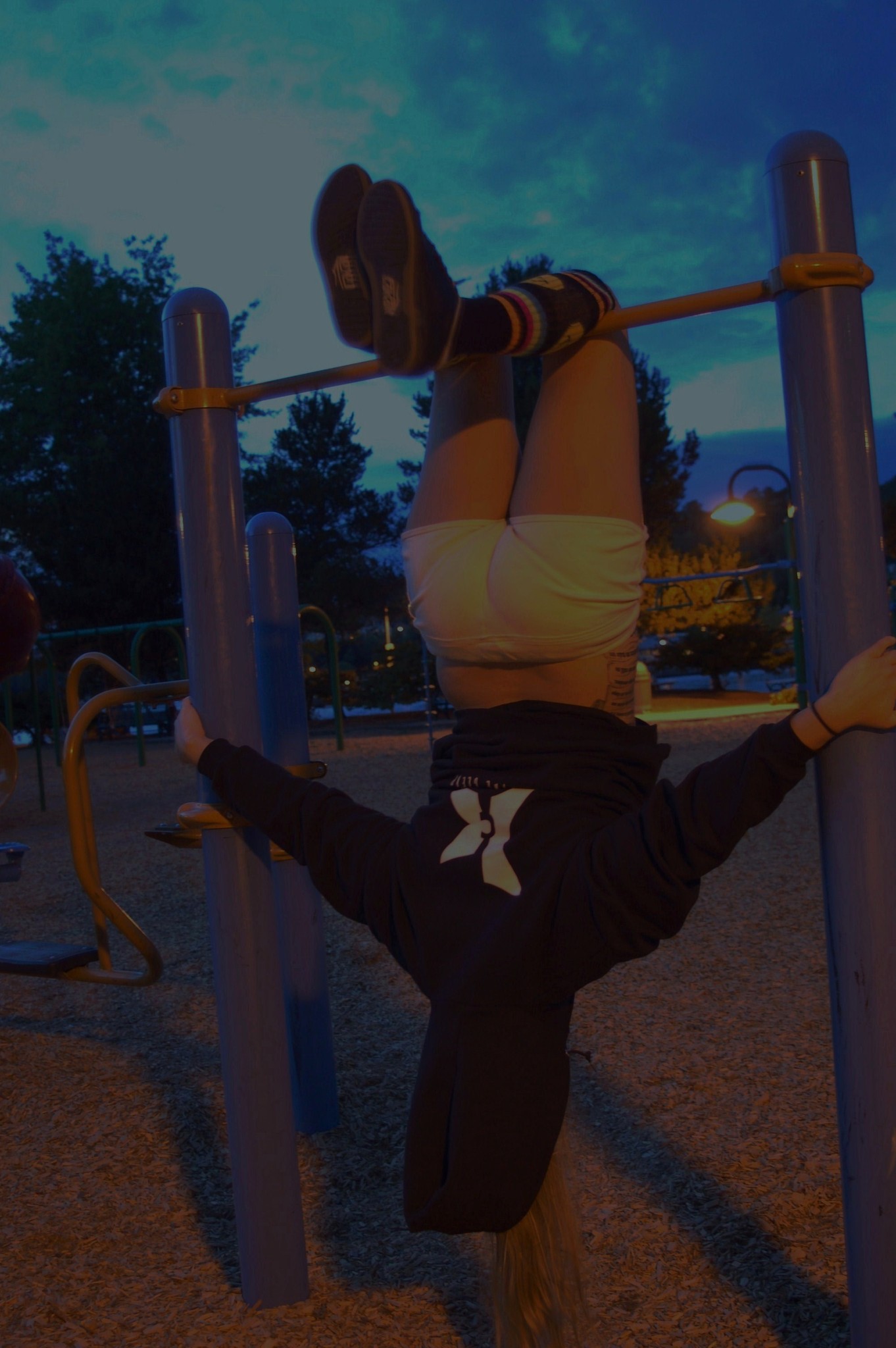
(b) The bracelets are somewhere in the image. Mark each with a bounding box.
[810,703,837,736]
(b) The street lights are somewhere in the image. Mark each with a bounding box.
[709,461,810,711]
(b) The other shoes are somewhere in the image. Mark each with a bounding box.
[356,181,459,375]
[312,163,376,349]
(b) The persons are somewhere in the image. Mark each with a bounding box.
[172,161,896,1347]
